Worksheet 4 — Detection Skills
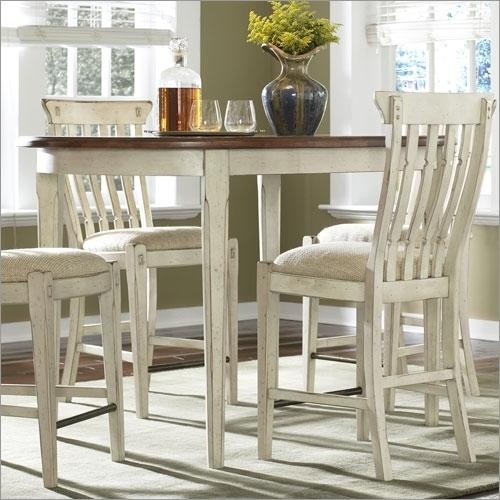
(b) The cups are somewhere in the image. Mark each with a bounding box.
[187,97,257,134]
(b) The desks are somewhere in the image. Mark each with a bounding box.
[10,128,450,470]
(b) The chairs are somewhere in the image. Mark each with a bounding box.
[1,248,128,489]
[297,92,496,403]
[254,92,496,485]
[38,93,239,422]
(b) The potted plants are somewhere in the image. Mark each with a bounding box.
[247,0,344,137]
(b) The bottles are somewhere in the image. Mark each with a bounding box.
[158,38,201,132]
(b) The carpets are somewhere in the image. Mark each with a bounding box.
[0,355,500,500]
